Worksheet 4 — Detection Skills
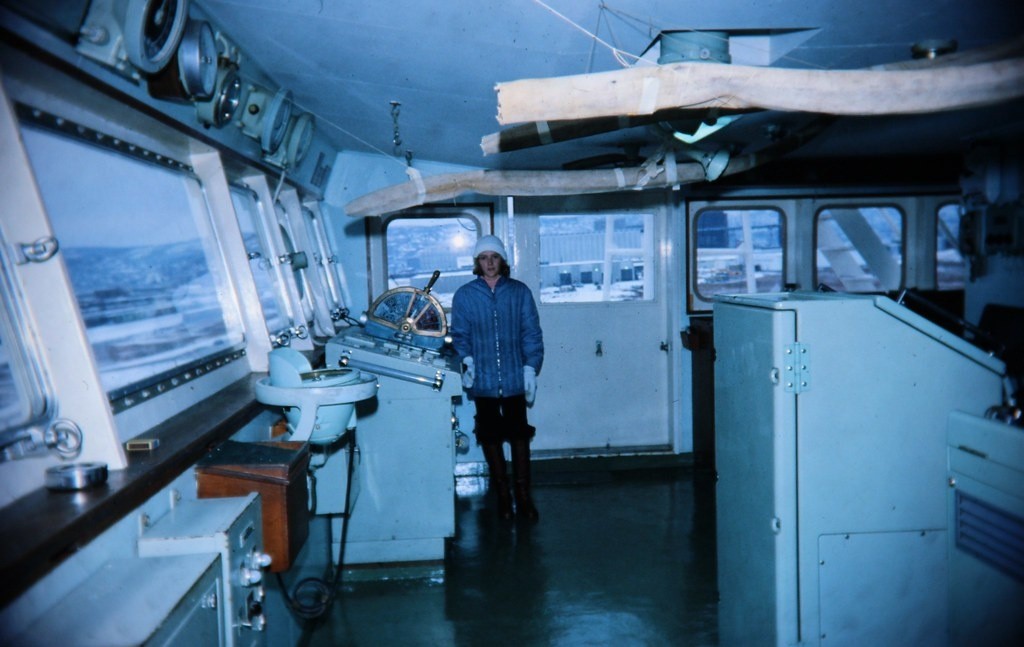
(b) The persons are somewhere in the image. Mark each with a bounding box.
[452,236,544,522]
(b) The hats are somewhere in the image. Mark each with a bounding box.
[473,234,507,261]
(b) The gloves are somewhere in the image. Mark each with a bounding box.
[522,366,537,403]
[461,355,476,389]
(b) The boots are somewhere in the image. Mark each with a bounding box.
[481,439,539,522]
